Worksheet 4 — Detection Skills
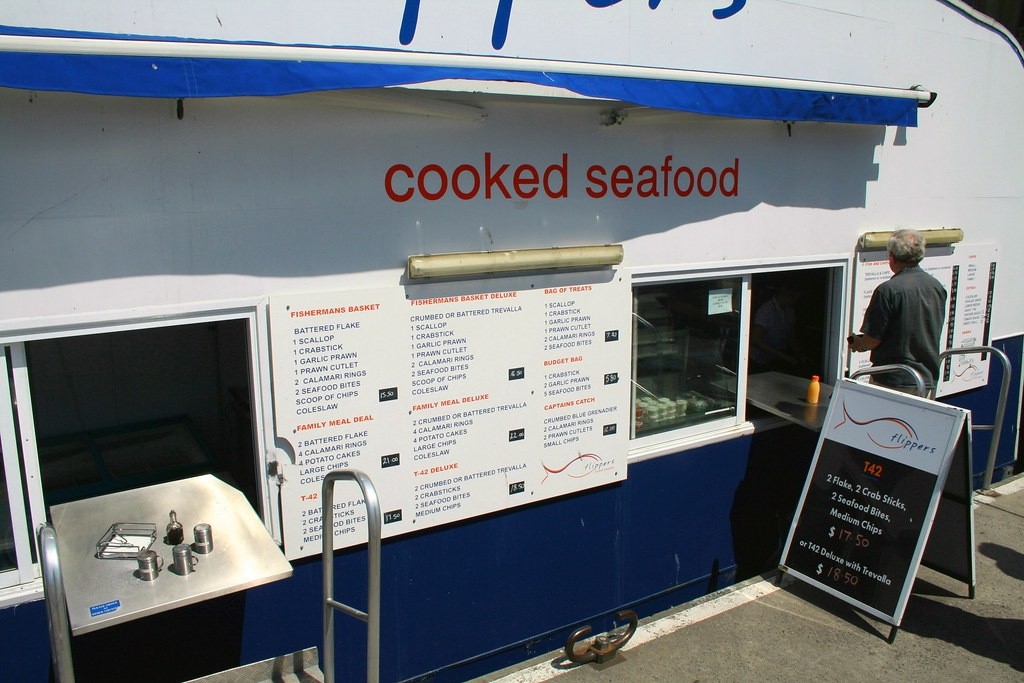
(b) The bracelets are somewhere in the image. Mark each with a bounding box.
[857,342,866,352]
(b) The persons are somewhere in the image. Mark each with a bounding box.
[751,279,817,377]
[848,229,947,398]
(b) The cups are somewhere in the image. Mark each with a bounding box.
[172,547,198,576]
[194,523,216,552]
[138,550,165,581]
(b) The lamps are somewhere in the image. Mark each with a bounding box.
[859,228,964,249]
[406,244,624,281]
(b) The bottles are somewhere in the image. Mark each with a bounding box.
[634,397,688,430]
[165,510,184,545]
[807,375,820,404]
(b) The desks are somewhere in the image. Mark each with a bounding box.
[712,371,834,433]
